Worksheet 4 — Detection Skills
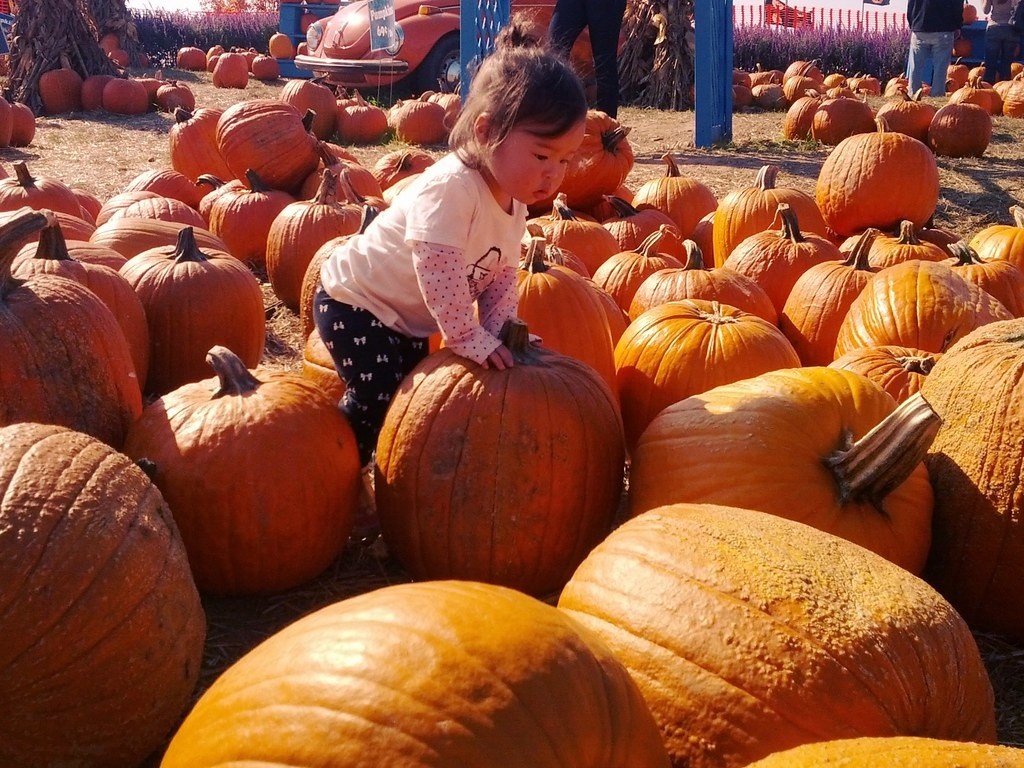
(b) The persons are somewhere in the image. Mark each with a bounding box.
[906,0,1024,98]
[548,0,627,120]
[311,22,587,493]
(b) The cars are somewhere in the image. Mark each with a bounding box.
[293,0,628,100]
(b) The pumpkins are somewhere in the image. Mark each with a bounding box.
[0,0,1024,768]
